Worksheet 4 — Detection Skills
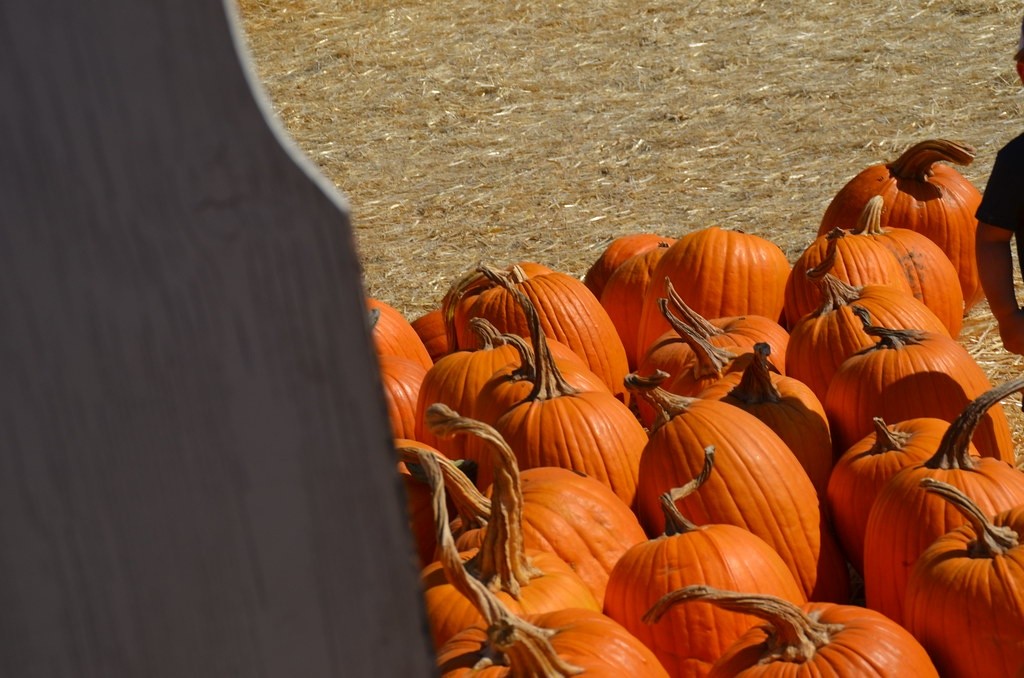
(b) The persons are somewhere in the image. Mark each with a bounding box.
[974,13,1024,412]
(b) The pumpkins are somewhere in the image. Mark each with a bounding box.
[365,136,1024,678]
